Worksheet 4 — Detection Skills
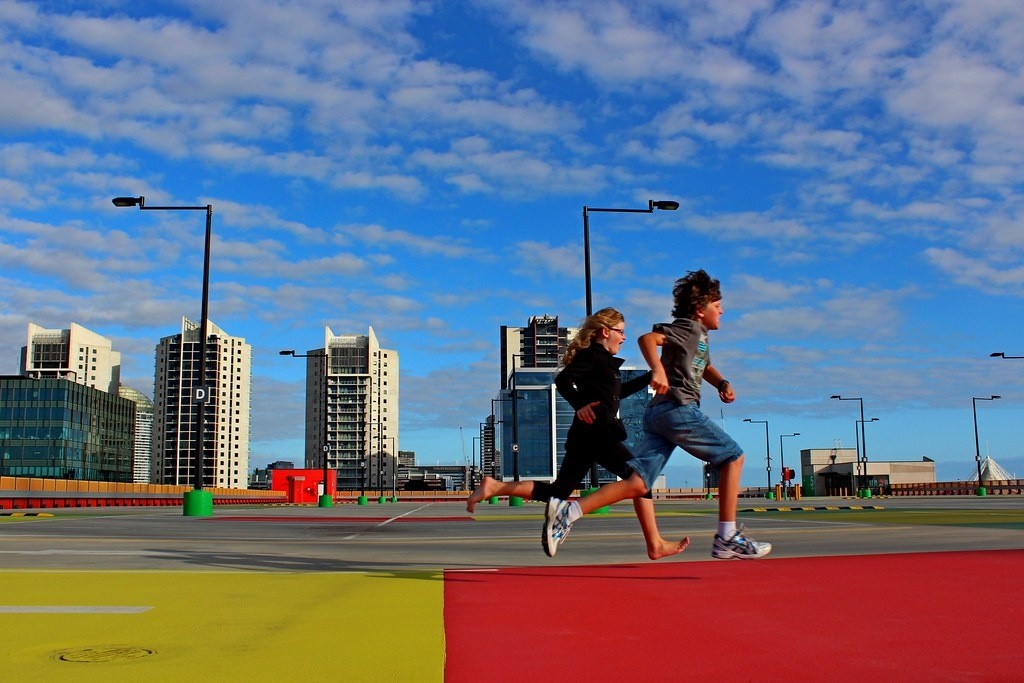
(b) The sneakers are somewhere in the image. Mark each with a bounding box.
[712,524,773,559]
[542,496,572,557]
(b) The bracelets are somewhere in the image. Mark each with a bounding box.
[718,380,729,392]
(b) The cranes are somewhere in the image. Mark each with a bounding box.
[460,427,470,490]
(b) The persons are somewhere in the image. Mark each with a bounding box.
[542,270,771,559]
[467,306,689,560]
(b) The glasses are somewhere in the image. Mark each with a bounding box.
[609,327,624,336]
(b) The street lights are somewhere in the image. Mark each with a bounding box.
[830,396,871,498]
[280,350,332,507]
[472,419,508,505]
[358,422,386,503]
[972,395,1002,496]
[510,348,562,507]
[781,433,800,496]
[332,395,367,505]
[584,200,680,513]
[373,436,397,503]
[112,197,213,519]
[744,419,773,500]
[489,396,526,504]
[855,418,881,497]
